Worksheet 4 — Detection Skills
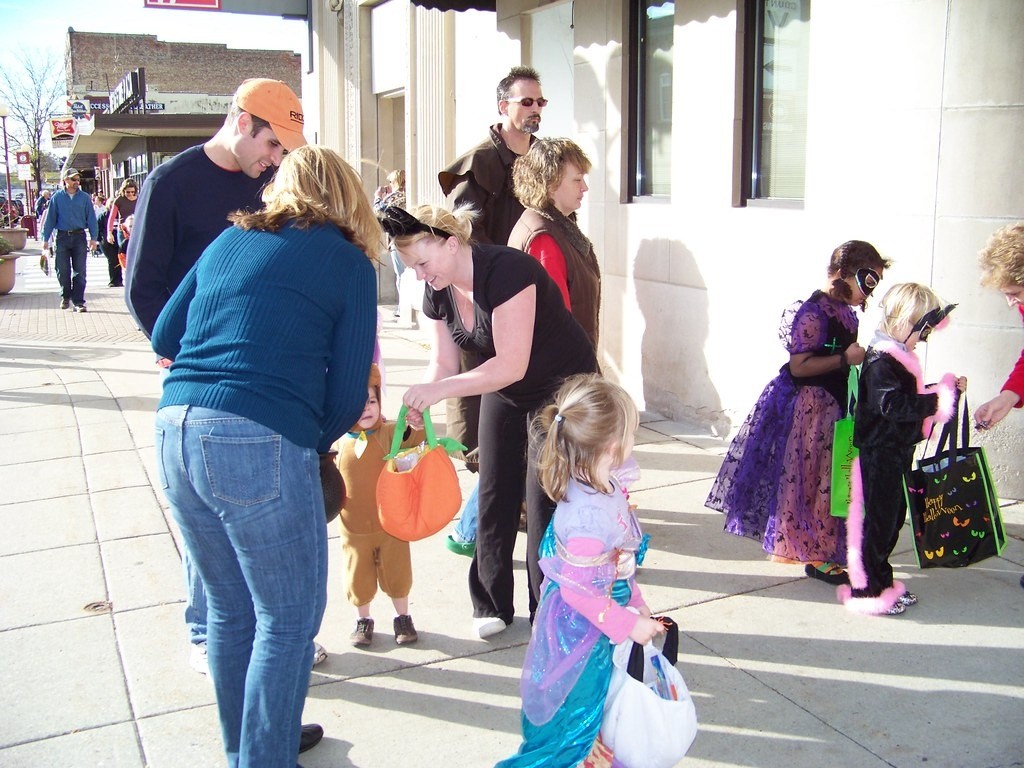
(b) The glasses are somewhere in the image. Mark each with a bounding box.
[506,97,548,107]
[69,177,80,181]
[125,189,136,193]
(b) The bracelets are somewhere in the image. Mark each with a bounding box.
[837,352,847,368]
[107,230,113,233]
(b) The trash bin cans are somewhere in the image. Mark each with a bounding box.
[21,215,37,237]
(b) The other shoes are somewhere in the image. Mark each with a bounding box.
[475,617,507,638]
[108,282,123,287]
[60,297,70,309]
[298,723,323,754]
[73,303,86,312]
[804,562,850,586]
[897,591,918,606]
[885,601,906,614]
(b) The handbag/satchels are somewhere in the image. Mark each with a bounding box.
[375,401,468,542]
[598,607,698,768]
[829,359,866,517]
[317,449,347,525]
[40,249,50,277]
[902,380,1008,569]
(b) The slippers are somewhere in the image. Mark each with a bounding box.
[446,535,477,558]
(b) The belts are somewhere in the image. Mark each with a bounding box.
[58,229,84,236]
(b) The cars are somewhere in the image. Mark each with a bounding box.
[0,188,25,225]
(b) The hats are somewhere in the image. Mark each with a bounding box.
[235,77,307,153]
[63,167,81,180]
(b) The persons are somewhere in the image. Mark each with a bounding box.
[41,168,99,313]
[125,77,327,666]
[972,222,1024,432]
[835,283,968,615]
[376,203,602,639]
[35,178,138,288]
[444,137,603,557]
[334,362,424,649]
[493,373,671,767]
[438,67,547,532]
[704,240,891,586]
[150,145,389,767]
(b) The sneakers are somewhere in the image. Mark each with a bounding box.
[313,643,327,665]
[189,639,209,674]
[349,618,374,647]
[394,614,417,645]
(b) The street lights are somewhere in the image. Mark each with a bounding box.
[0,103,15,229]
